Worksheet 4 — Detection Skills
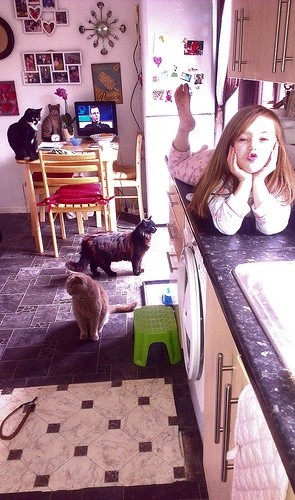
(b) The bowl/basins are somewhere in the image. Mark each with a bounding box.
[89,133,117,143]
[70,138,83,146]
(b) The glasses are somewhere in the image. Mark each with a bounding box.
[91,112,100,115]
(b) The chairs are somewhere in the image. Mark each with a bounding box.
[38,147,116,258]
[22,173,74,236]
[96,127,145,227]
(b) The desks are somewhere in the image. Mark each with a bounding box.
[16,138,119,253]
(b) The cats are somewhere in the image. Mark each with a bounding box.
[65,270,139,340]
[41,104,66,141]
[65,215,157,277]
[7,107,43,162]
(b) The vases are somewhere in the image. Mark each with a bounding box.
[62,127,74,142]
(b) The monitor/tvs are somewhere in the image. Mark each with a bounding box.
[75,101,118,136]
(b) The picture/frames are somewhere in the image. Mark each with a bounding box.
[22,51,83,86]
[13,0,68,36]
[92,62,124,105]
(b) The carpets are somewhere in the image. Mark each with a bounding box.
[0,376,193,493]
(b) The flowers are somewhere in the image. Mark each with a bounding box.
[54,88,77,136]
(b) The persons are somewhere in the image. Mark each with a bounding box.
[27,55,79,83]
[195,74,202,84]
[84,106,110,129]
[168,83,295,235]
[26,20,39,32]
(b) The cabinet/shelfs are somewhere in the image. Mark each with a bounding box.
[167,175,295,500]
[227,0,295,83]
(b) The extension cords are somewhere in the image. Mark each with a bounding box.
[121,205,139,216]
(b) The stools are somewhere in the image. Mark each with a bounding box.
[132,305,182,367]
